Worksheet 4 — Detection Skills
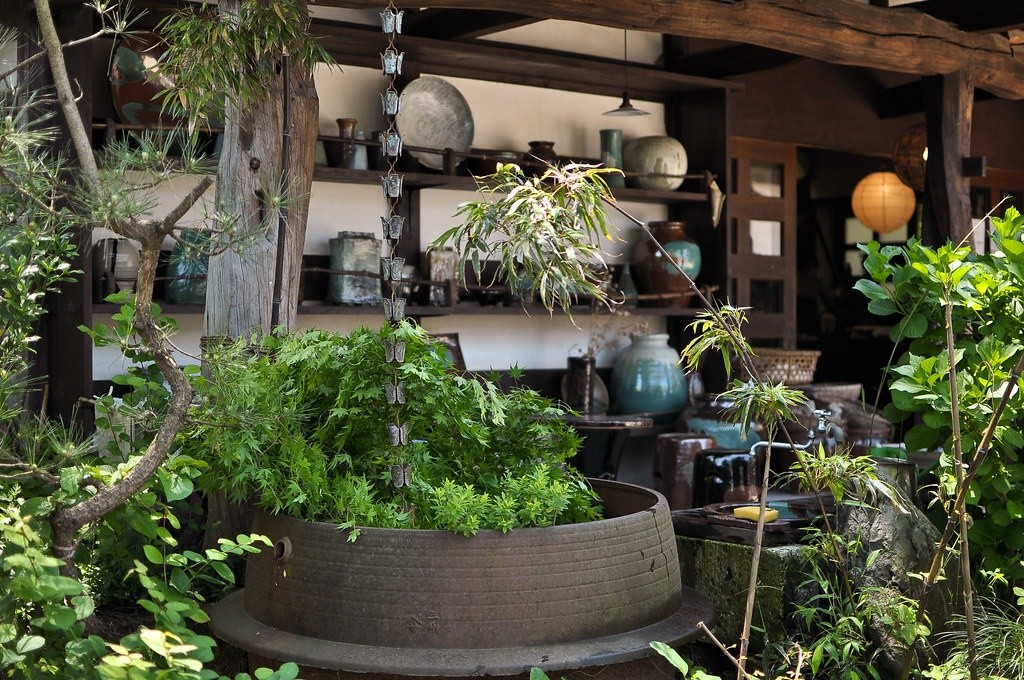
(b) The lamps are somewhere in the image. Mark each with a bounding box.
[602,30,650,116]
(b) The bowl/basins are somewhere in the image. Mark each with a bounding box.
[483,149,523,177]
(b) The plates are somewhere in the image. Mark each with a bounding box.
[561,372,609,417]
[395,76,474,170]
[424,333,467,379]
[110,32,191,144]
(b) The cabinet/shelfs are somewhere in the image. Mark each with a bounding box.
[28,17,733,459]
